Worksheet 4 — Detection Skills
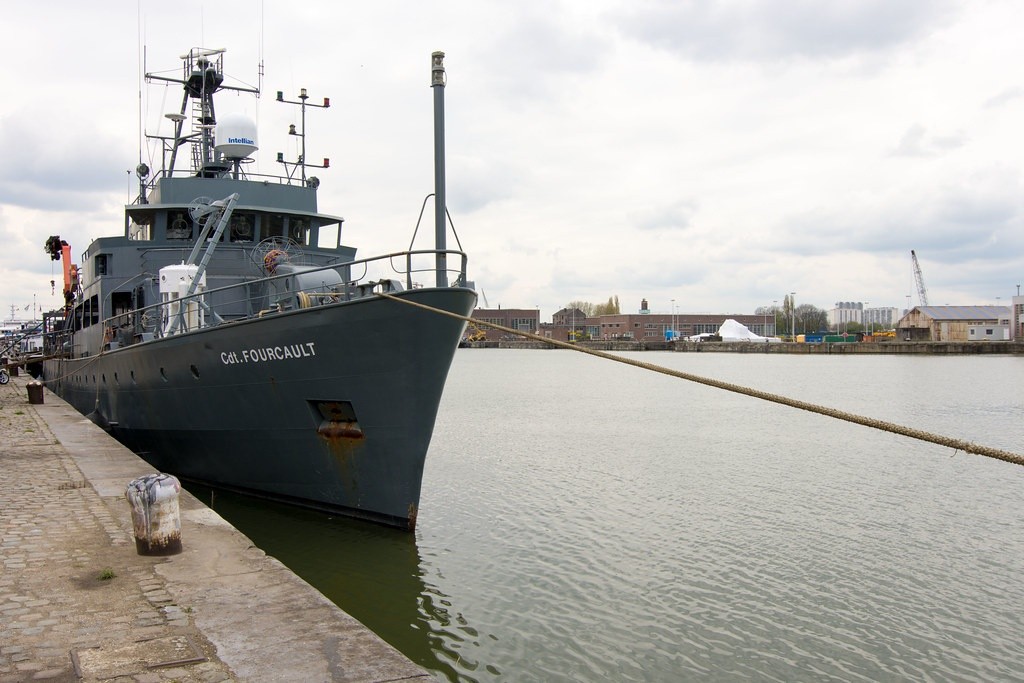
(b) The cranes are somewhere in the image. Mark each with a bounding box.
[911,248,928,308]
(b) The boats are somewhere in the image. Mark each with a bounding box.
[43,47,480,546]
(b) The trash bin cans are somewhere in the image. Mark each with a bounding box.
[25,380,44,404]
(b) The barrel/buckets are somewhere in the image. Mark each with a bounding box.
[117,328,135,348]
[110,341,119,350]
[665,331,673,341]
